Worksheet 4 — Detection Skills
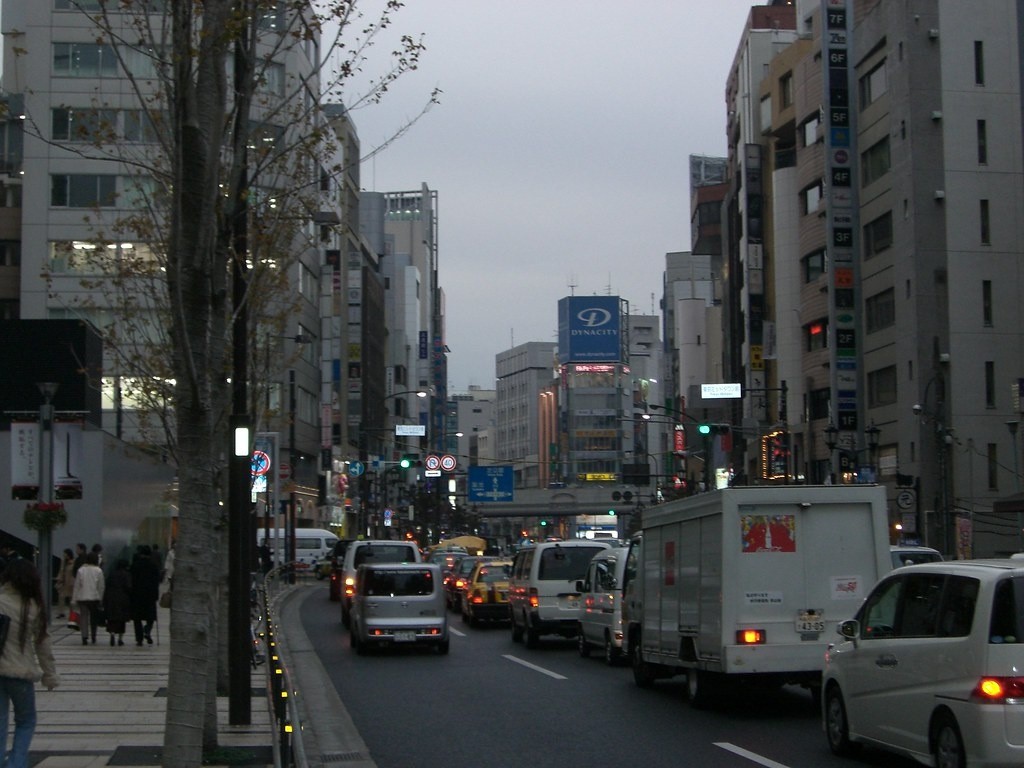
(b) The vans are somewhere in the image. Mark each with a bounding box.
[336,538,422,624]
[256,527,342,572]
[819,549,1024,768]
[502,538,615,651]
[889,546,945,571]
[574,546,641,663]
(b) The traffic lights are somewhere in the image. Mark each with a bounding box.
[401,460,423,468]
[697,422,730,435]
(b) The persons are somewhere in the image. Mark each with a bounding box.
[164,541,176,592]
[0,558,62,768]
[70,552,105,645]
[91,543,103,567]
[54,549,75,618]
[259,538,275,580]
[103,544,166,647]
[72,543,89,578]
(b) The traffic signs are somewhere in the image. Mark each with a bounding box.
[468,464,514,501]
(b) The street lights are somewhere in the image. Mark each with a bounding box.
[425,432,464,458]
[375,391,427,459]
[624,449,658,505]
[264,330,314,569]
[649,404,710,491]
[641,414,688,484]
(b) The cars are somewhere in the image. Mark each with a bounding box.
[314,533,502,613]
[344,560,451,655]
[454,561,514,628]
[54,475,82,499]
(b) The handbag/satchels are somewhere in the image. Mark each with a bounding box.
[67,602,81,632]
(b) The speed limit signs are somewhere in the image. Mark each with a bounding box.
[440,455,457,472]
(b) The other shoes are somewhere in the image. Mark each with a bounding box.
[136,642,142,645]
[110,636,115,646]
[56,614,65,619]
[144,634,153,644]
[83,641,87,645]
[118,640,123,645]
[92,638,95,643]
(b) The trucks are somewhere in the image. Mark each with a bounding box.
[595,481,894,708]
